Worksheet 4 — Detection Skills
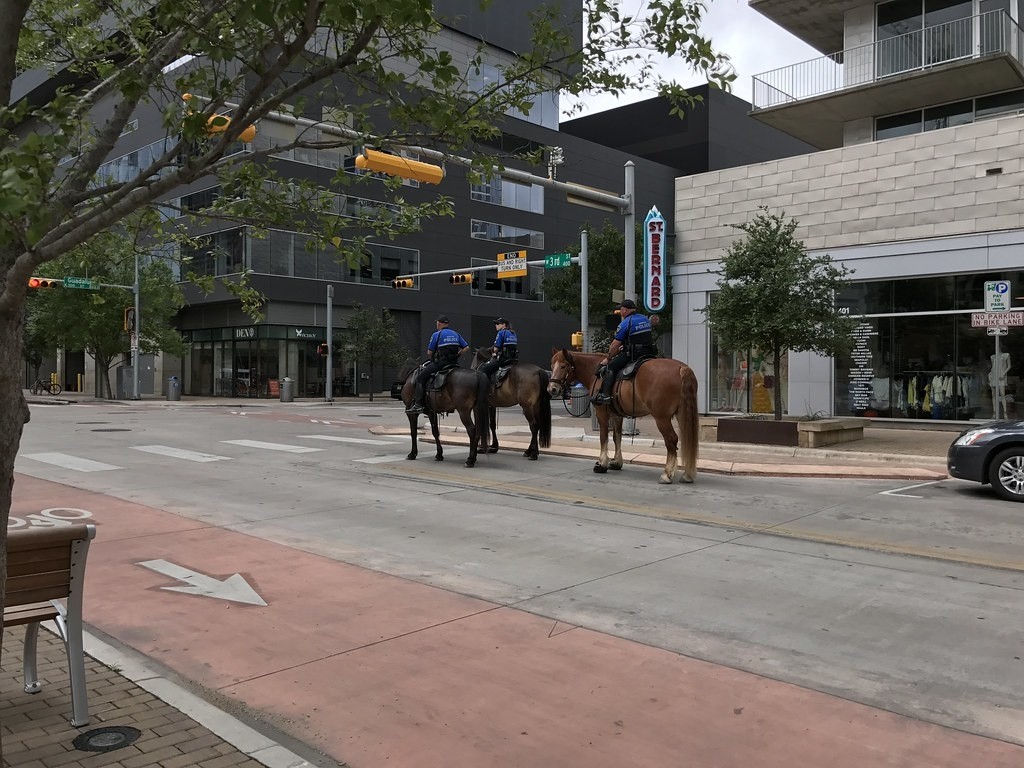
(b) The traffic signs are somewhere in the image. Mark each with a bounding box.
[545,253,571,269]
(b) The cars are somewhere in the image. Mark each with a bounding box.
[563,381,579,400]
[220,368,250,386]
[946,419,1024,503]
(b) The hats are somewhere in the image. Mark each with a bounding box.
[616,299,636,309]
[493,318,509,324]
[433,316,449,323]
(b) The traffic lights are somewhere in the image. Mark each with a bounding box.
[29,278,57,289]
[355,149,442,185]
[317,345,328,354]
[392,279,413,289]
[572,334,582,346]
[605,314,622,331]
[449,274,472,285]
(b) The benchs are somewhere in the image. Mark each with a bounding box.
[2,523,97,728]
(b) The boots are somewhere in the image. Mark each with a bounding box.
[590,369,616,403]
[405,383,424,413]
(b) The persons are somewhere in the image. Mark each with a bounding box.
[719,349,788,414]
[476,318,519,375]
[989,352,1011,419]
[590,299,652,405]
[403,315,469,414]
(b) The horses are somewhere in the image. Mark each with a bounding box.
[545,347,699,485]
[469,346,552,460]
[397,356,492,469]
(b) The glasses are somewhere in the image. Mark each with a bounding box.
[494,323,500,325]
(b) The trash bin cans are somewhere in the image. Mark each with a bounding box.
[166,376,182,401]
[589,402,613,431]
[570,386,591,418]
[279,377,295,402]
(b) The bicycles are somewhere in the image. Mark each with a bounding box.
[30,379,62,395]
